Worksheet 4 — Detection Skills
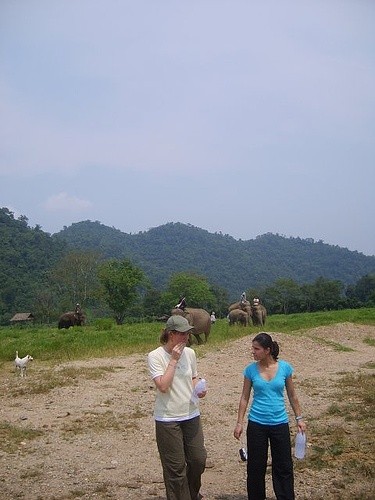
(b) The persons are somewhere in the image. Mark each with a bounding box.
[74,304,82,326]
[147,315,207,500]
[241,292,262,306]
[174,295,187,310]
[211,312,216,324]
[233,333,306,500]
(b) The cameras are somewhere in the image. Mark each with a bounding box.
[239,447,248,461]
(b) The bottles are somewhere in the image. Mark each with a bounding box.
[191,378,205,404]
[295,430,305,458]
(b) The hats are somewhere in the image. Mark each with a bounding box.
[166,315,194,332]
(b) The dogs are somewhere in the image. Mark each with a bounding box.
[15,351,34,376]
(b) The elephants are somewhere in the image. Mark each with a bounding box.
[228,300,266,326]
[171,308,210,346]
[58,308,84,329]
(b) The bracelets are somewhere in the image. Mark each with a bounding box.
[295,417,302,421]
[168,364,177,368]
